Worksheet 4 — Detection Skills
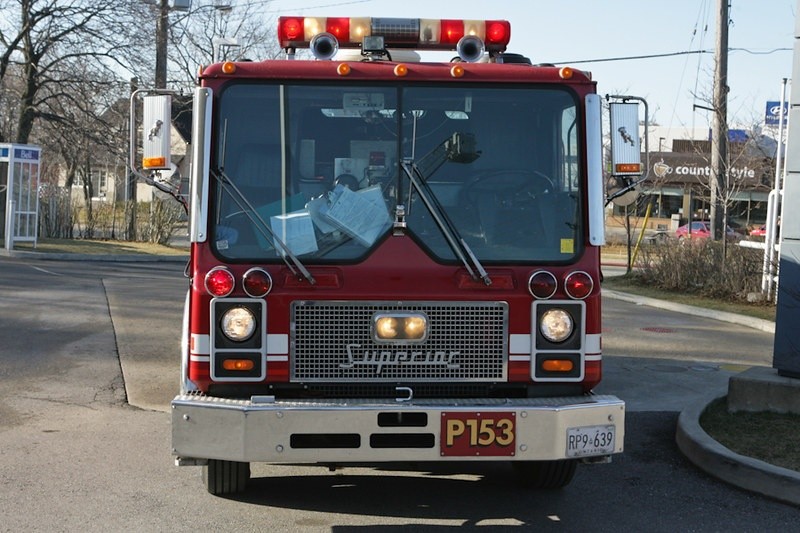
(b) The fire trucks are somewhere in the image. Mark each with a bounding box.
[127,13,650,498]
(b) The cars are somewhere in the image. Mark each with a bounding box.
[749,223,780,240]
[675,220,743,244]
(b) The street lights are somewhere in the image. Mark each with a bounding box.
[658,137,666,219]
